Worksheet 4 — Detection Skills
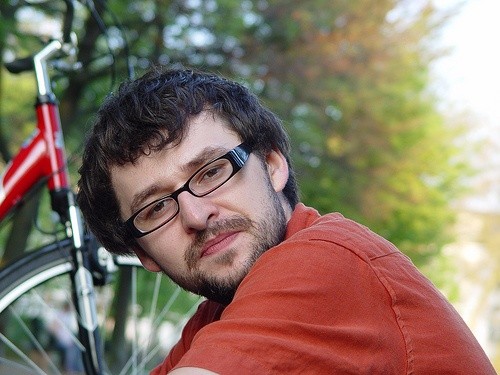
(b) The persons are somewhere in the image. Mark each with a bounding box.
[30,283,85,375]
[74,65,499,375]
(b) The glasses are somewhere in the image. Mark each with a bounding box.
[124,143,255,239]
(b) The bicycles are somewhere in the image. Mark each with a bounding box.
[2,1,219,374]
